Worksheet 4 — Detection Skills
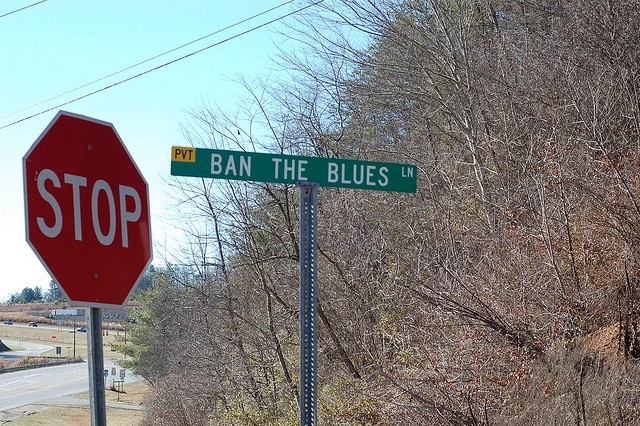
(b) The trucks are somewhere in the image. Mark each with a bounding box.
[50,309,81,317]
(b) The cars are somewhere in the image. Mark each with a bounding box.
[76,326,86,332]
[4,318,12,325]
[28,321,38,327]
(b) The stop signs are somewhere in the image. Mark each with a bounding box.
[22,109,155,306]
